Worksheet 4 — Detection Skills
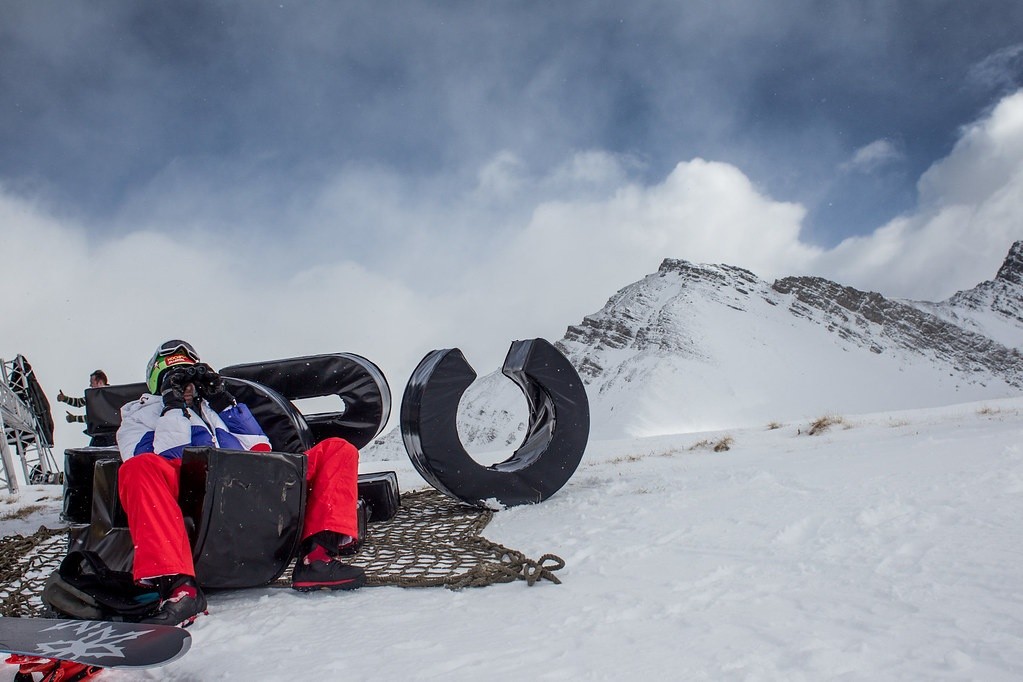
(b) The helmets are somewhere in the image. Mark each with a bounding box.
[145,339,201,394]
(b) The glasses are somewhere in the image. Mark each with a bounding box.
[159,339,200,362]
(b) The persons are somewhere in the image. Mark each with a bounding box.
[116,340,365,627]
[58,370,109,423]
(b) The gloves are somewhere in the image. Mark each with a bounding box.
[187,363,235,414]
[159,368,195,409]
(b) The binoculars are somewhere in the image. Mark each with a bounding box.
[183,365,207,383]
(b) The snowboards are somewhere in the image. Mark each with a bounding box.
[0,617,190,668]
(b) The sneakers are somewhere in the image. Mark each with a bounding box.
[140,574,208,628]
[292,545,363,590]
